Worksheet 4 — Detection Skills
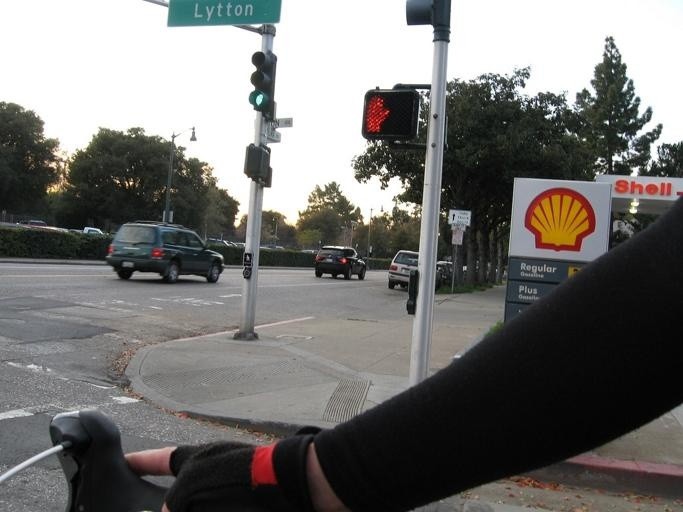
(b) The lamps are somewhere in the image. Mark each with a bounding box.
[628,199,639,215]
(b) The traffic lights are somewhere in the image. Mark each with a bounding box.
[248,48,277,120]
[361,88,420,140]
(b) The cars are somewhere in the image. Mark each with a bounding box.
[389,249,453,291]
[28,220,103,234]
[207,238,245,247]
[314,245,366,280]
[105,220,225,283]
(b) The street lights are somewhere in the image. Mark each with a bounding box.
[164,126,197,222]
[272,217,279,246]
[365,204,384,270]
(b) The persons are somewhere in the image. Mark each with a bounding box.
[121,192,683,512]
